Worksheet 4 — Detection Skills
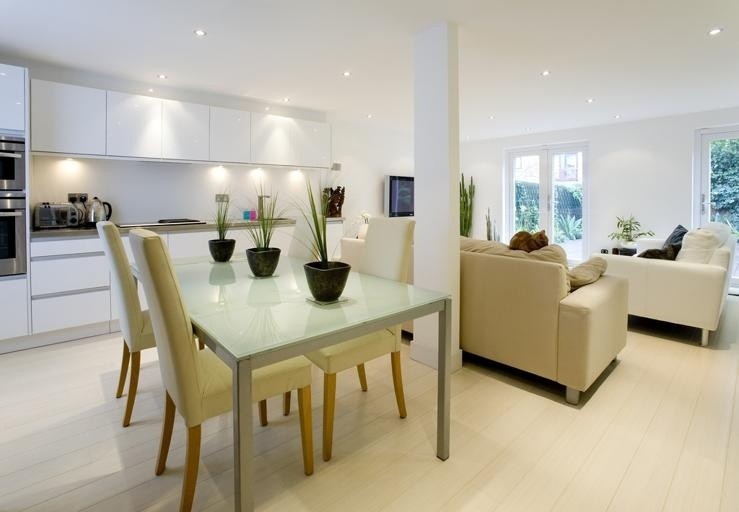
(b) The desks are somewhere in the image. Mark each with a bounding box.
[125,252,452,512]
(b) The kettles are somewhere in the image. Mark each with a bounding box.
[84,196,112,226]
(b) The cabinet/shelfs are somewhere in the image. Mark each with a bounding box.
[30,237,109,347]
[289,119,331,169]
[1,65,26,132]
[162,101,210,161]
[2,277,30,339]
[170,227,291,266]
[109,232,168,332]
[252,113,290,164]
[29,79,107,156]
[210,107,251,164]
[107,90,164,160]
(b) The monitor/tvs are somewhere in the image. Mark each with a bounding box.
[384,175,414,218]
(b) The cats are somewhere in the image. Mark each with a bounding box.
[508,229,548,253]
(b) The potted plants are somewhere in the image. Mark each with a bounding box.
[208,188,235,262]
[608,215,655,248]
[303,175,352,302]
[245,182,283,277]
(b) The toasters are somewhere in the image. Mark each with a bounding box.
[32,202,80,230]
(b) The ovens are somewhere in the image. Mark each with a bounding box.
[0,138,27,279]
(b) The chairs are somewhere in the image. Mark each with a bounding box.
[95,222,205,428]
[129,229,313,512]
[592,222,736,346]
[306,218,416,461]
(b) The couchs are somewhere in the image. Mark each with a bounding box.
[338,235,629,405]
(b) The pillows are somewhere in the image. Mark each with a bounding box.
[663,224,687,246]
[569,257,607,286]
